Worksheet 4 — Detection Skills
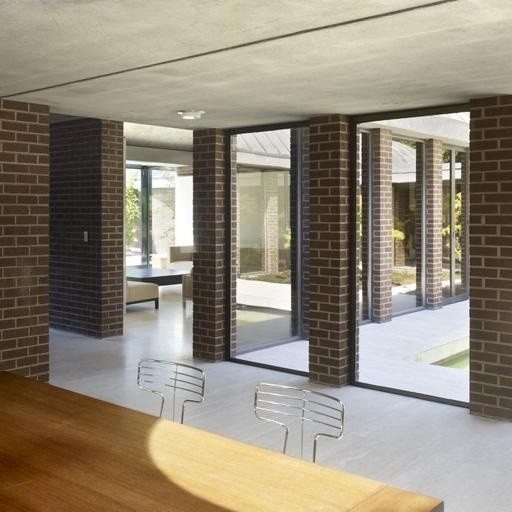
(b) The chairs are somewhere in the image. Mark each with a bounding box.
[181,267,193,309]
[137,357,346,462]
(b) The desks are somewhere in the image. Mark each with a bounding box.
[0,369,444,511]
